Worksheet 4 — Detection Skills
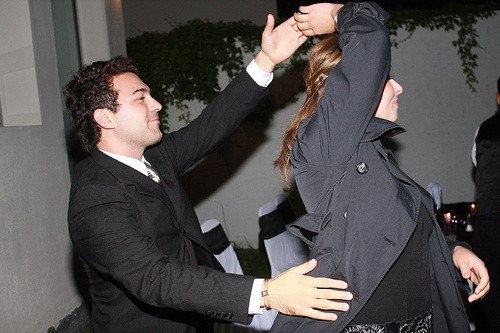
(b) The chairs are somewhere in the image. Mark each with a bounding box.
[200,194,311,333]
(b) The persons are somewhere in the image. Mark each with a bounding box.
[268,0,492,333]
[62,11,353,333]
[471,76,500,275]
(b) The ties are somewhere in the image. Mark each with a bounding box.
[144,162,161,183]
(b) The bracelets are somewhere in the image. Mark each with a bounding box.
[260,276,271,310]
[332,10,340,31]
